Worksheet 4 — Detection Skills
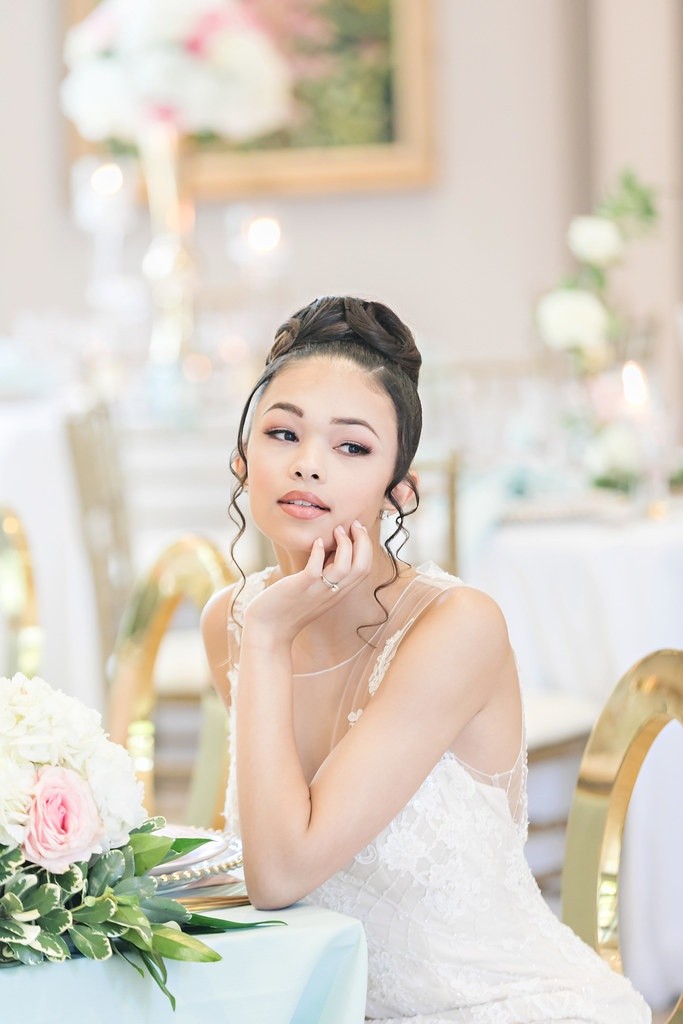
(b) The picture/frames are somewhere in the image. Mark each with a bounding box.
[63,0,441,200]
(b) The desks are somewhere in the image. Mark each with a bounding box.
[428,506,683,745]
[0,902,368,1024]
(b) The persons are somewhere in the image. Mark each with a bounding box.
[200,296,652,1024]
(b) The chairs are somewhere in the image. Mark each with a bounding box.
[102,537,241,825]
[63,400,229,778]
[560,646,683,1023]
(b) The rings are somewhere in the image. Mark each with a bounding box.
[321,575,339,592]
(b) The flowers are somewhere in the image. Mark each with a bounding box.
[0,672,287,1013]
